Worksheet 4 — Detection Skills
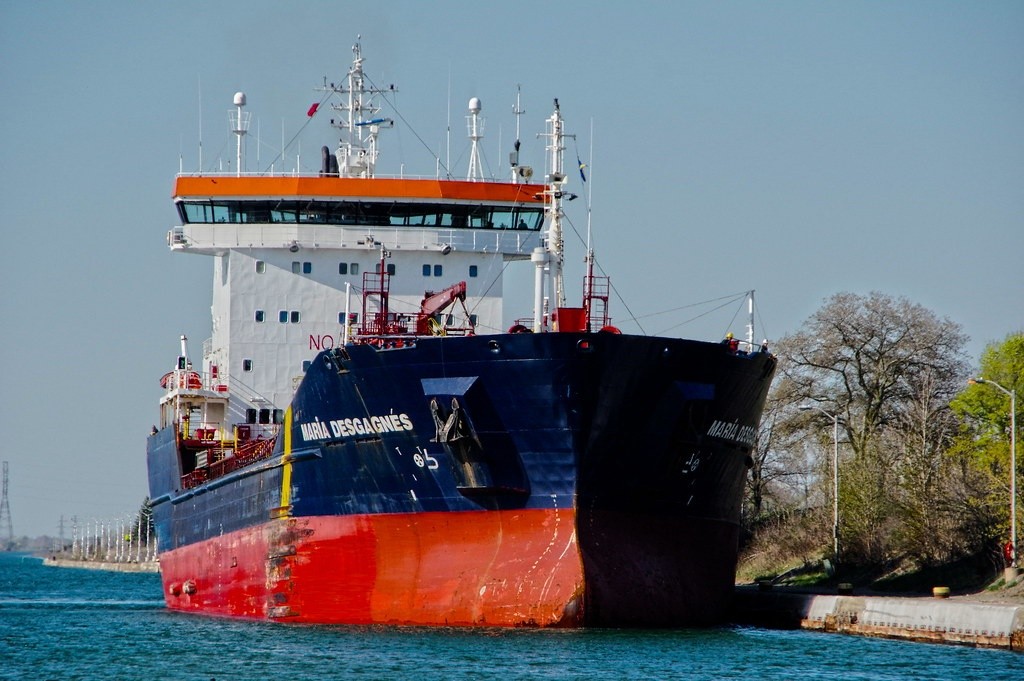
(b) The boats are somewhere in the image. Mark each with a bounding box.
[146,35,778,631]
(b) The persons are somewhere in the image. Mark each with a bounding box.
[720,332,733,344]
[761,338,768,352]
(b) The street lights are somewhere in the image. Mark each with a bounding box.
[968,378,1024,584]
[81,517,125,561]
[135,513,142,561]
[143,512,150,561]
[798,405,840,571]
[126,513,132,561]
